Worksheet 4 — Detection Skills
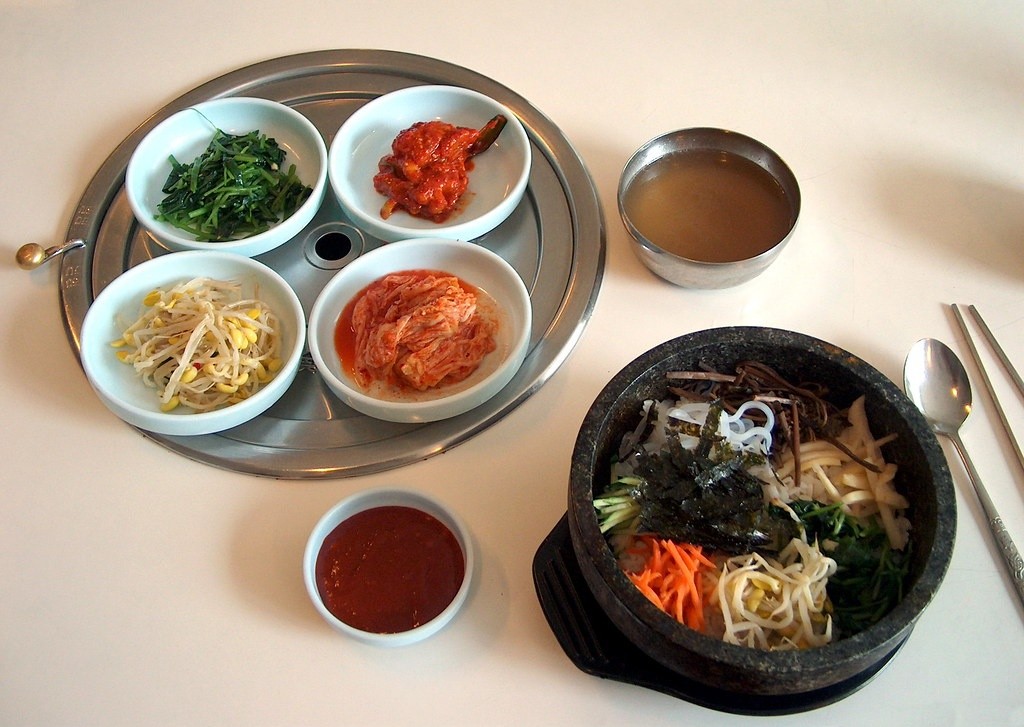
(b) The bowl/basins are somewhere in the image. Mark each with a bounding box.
[567,325,956,693]
[307,237,533,424]
[617,126,802,290]
[328,84,531,241]
[124,97,327,258]
[80,250,306,436]
[302,487,475,648]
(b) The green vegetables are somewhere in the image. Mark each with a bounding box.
[768,495,915,637]
[152,127,315,243]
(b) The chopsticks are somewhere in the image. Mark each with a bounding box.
[950,303,1024,469]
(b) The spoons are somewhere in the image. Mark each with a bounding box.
[903,337,1024,603]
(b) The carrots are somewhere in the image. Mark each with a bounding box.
[617,535,720,637]
